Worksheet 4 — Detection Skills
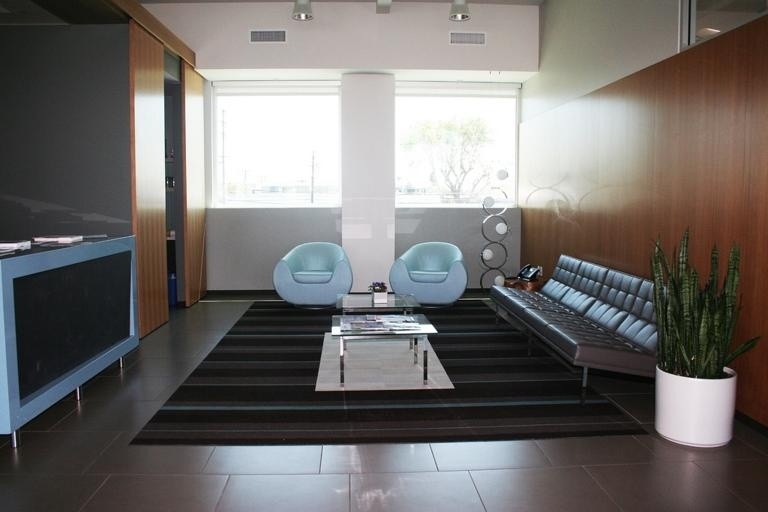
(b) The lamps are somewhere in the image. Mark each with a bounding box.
[447,0,471,21]
[290,0,316,21]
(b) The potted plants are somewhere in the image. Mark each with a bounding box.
[647,226,764,447]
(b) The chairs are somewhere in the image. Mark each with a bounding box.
[388,241,467,307]
[270,241,354,310]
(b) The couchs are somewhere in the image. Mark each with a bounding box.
[488,253,669,388]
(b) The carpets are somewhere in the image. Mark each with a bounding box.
[314,331,456,392]
[128,299,650,447]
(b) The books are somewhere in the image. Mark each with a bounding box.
[338,313,422,334]
[34,232,84,244]
[1,239,32,252]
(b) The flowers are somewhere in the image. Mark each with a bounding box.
[367,281,386,292]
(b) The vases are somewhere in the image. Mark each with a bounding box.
[372,292,386,304]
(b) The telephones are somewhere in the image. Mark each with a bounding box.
[519,264,539,281]
